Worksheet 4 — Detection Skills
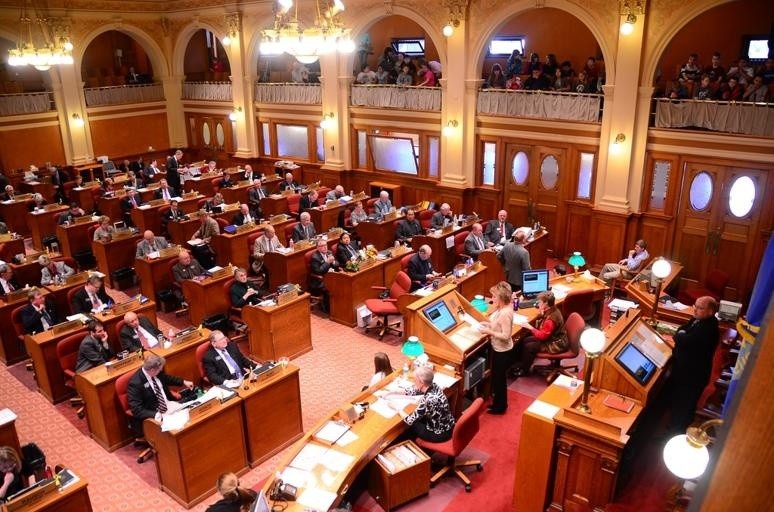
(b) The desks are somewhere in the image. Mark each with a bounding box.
[0,156,549,511]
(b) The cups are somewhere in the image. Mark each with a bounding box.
[565,275,572,283]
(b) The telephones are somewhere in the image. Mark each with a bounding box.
[270,479,297,501]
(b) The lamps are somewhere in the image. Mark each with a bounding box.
[577,329,607,413]
[644,259,671,327]
[7,1,76,73]
[222,10,242,45]
[662,419,728,479]
[259,0,356,64]
[616,0,645,34]
[438,0,466,37]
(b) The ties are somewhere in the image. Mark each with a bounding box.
[92,294,97,304]
[151,377,167,413]
[136,328,149,350]
[222,351,243,378]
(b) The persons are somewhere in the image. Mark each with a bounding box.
[668,51,769,102]
[598,239,650,282]
[666,296,719,430]
[125,67,140,85]
[487,50,595,93]
[292,47,442,86]
[1,150,569,512]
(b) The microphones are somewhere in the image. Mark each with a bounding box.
[457,301,494,315]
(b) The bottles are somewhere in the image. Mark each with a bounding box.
[0,159,357,512]
[569,375,579,396]
[513,297,519,311]
[403,363,409,380]
[358,192,487,278]
[433,280,438,290]
[534,222,540,230]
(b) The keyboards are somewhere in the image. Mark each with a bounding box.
[517,299,538,310]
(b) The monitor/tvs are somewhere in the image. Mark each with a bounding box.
[614,341,657,386]
[521,268,550,299]
[422,299,457,333]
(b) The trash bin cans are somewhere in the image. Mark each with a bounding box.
[21,443,45,483]
[202,313,227,335]
[74,251,94,270]
[111,267,134,291]
[157,290,177,313]
[42,235,56,250]
